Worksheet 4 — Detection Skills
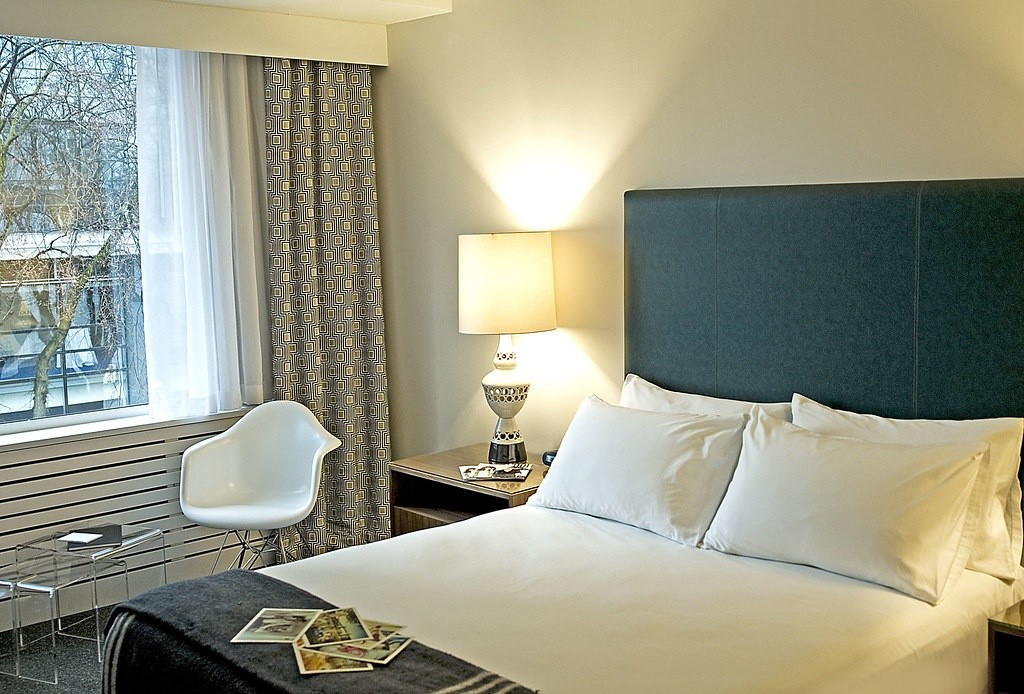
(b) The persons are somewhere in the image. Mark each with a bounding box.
[463,468,476,480]
[472,465,496,478]
[492,464,529,480]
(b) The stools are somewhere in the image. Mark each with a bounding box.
[0,552,130,685]
[15,519,168,664]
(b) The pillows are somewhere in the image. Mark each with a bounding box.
[697,404,993,608]
[790,393,1024,583]
[619,374,793,424]
[526,392,751,549]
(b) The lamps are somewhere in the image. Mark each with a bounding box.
[458,231,559,465]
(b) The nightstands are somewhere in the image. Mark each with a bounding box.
[988,599,1024,694]
[387,441,556,539]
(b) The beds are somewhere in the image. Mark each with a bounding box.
[101,178,1024,694]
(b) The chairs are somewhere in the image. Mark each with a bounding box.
[179,400,343,578]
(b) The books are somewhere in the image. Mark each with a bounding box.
[465,462,533,481]
[458,465,477,481]
[58,524,164,562]
